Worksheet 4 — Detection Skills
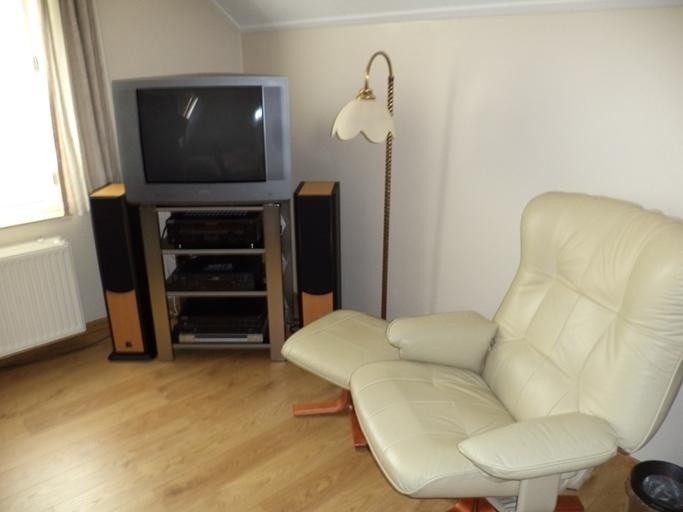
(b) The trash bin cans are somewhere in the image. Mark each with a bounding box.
[623,459,683,512]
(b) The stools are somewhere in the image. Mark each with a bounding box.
[281,307,399,450]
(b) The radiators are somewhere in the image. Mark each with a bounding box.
[0,235,88,359]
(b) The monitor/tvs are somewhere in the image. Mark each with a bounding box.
[110,74,291,202]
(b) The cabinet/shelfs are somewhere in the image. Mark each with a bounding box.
[140,200,295,362]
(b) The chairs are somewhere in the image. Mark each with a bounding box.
[350,189,682,511]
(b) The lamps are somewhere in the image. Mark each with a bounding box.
[331,51,394,319]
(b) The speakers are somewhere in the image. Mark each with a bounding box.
[88,182,158,362]
[293,181,342,328]
